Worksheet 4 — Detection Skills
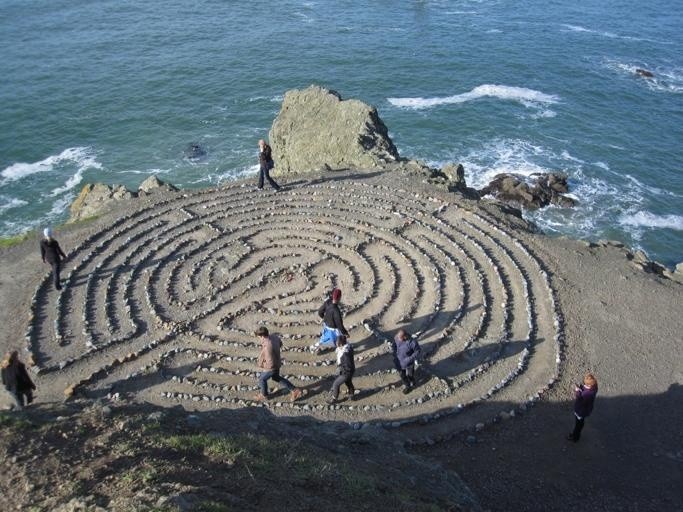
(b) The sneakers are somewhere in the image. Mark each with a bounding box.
[289,388,301,401]
[254,393,268,401]
[325,394,355,404]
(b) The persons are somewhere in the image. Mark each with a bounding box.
[37,224,68,293]
[310,289,352,354]
[253,326,302,404]
[253,137,283,194]
[565,372,598,443]
[326,333,355,406]
[0,348,38,411]
[386,328,422,394]
